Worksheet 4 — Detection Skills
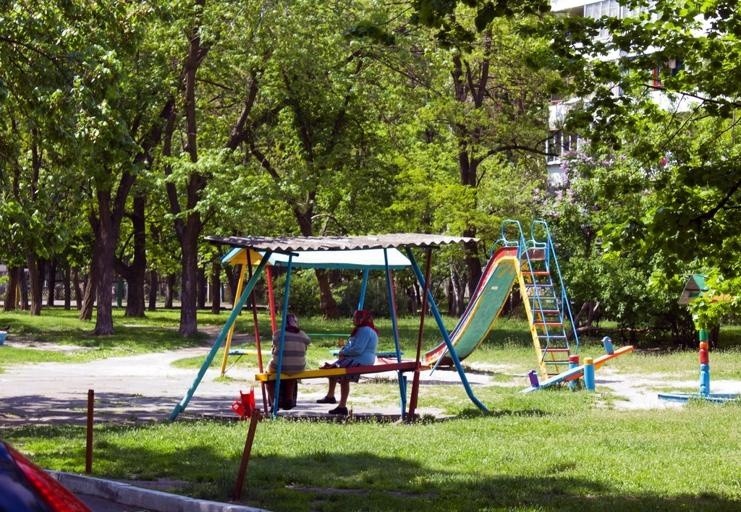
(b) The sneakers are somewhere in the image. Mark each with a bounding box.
[317,396,337,403]
[329,407,348,415]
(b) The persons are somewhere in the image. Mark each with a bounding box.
[265,314,311,410]
[315,310,378,413]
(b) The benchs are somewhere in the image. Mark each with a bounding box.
[255,350,419,416]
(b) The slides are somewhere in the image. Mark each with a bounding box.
[425,245,545,367]
